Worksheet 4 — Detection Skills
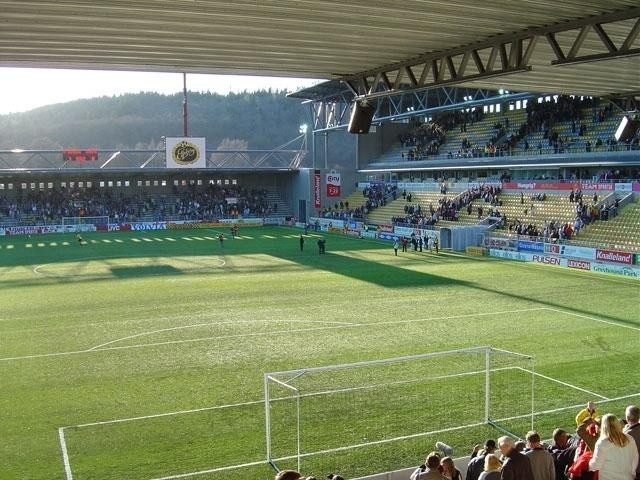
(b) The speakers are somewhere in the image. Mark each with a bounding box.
[614,116,632,142]
[347,102,377,134]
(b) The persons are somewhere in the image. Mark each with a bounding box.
[300,236,304,253]
[317,238,326,255]
[509,167,627,239]
[399,94,634,161]
[393,169,509,254]
[408,399,640,479]
[2,172,297,249]
[328,221,381,232]
[303,221,320,235]
[318,184,398,220]
